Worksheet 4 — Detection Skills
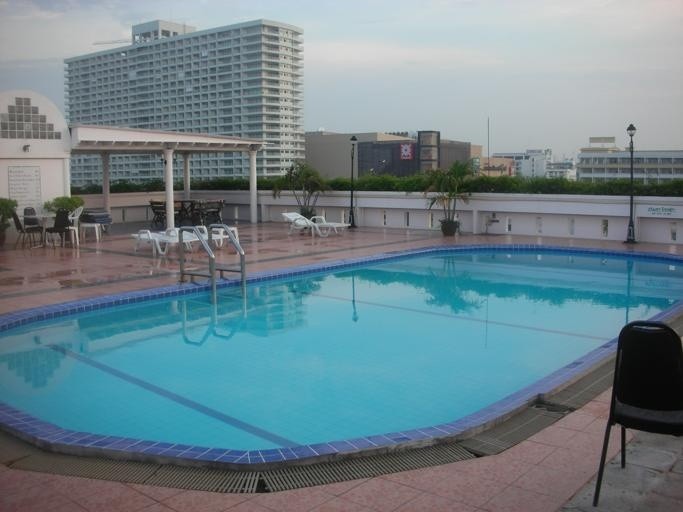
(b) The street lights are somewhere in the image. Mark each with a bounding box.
[346,134,360,229]
[623,123,641,245]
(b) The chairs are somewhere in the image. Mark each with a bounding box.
[13,206,101,250]
[149,199,225,230]
[281,211,352,237]
[130,224,239,256]
[593,322,681,506]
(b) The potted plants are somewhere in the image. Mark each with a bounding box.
[44,196,85,241]
[0,198,18,248]
[424,156,485,236]
[272,159,333,228]
